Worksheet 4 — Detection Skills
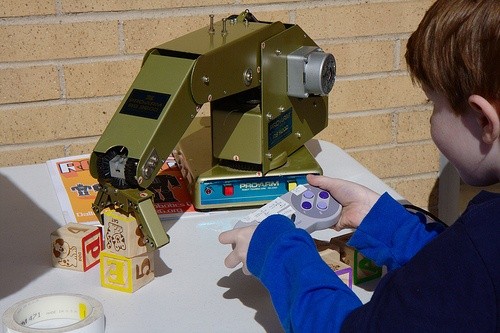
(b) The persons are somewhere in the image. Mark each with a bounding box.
[219,0,500,332]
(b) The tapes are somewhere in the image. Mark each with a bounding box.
[3,293,105,333]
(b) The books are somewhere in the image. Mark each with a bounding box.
[46,151,209,226]
